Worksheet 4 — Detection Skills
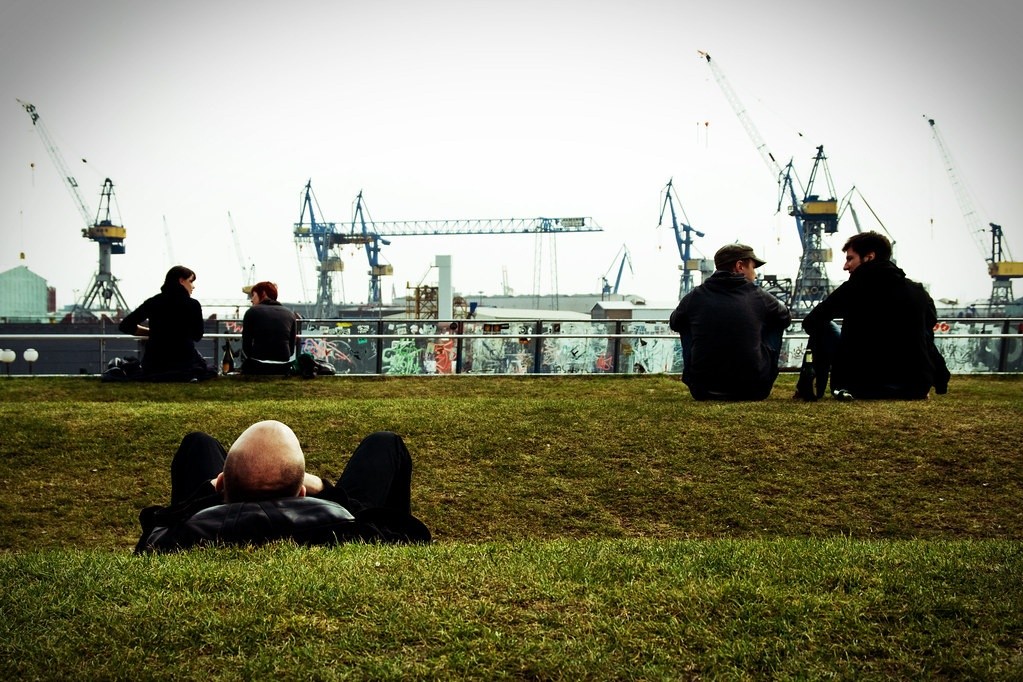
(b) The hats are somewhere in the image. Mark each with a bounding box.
[714,244,766,271]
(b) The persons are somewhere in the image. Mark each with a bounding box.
[239,283,296,376]
[119,266,219,380]
[793,231,950,402]
[670,244,791,402]
[142,421,431,545]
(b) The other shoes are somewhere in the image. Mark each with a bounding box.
[793,391,818,402]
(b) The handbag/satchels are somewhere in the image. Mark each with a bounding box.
[103,356,139,382]
[298,353,336,380]
[185,495,355,549]
